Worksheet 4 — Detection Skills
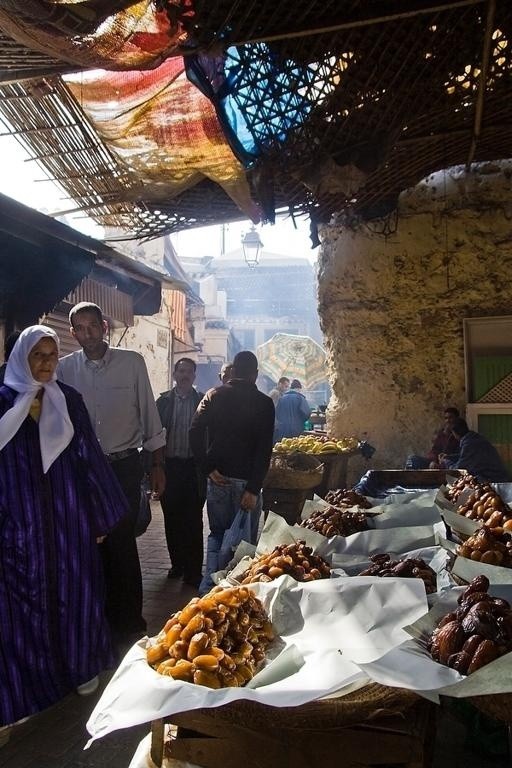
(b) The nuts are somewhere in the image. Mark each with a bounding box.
[457,484,512,568]
[146,540,330,690]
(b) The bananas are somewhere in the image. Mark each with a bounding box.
[319,440,342,454]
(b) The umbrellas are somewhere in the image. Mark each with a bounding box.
[256,333,329,392]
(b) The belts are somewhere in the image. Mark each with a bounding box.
[106,449,139,463]
[167,458,193,465]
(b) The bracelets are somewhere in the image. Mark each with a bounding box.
[152,462,165,467]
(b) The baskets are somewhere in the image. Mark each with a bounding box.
[264,451,325,489]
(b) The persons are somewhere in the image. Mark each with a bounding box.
[147,358,207,588]
[406,408,508,481]
[271,376,311,441]
[0,330,22,388]
[56,301,168,632]
[0,324,131,728]
[189,350,275,588]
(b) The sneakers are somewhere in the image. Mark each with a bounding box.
[184,573,203,589]
[168,563,185,578]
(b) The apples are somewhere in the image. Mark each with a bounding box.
[274,433,318,455]
[337,437,359,451]
